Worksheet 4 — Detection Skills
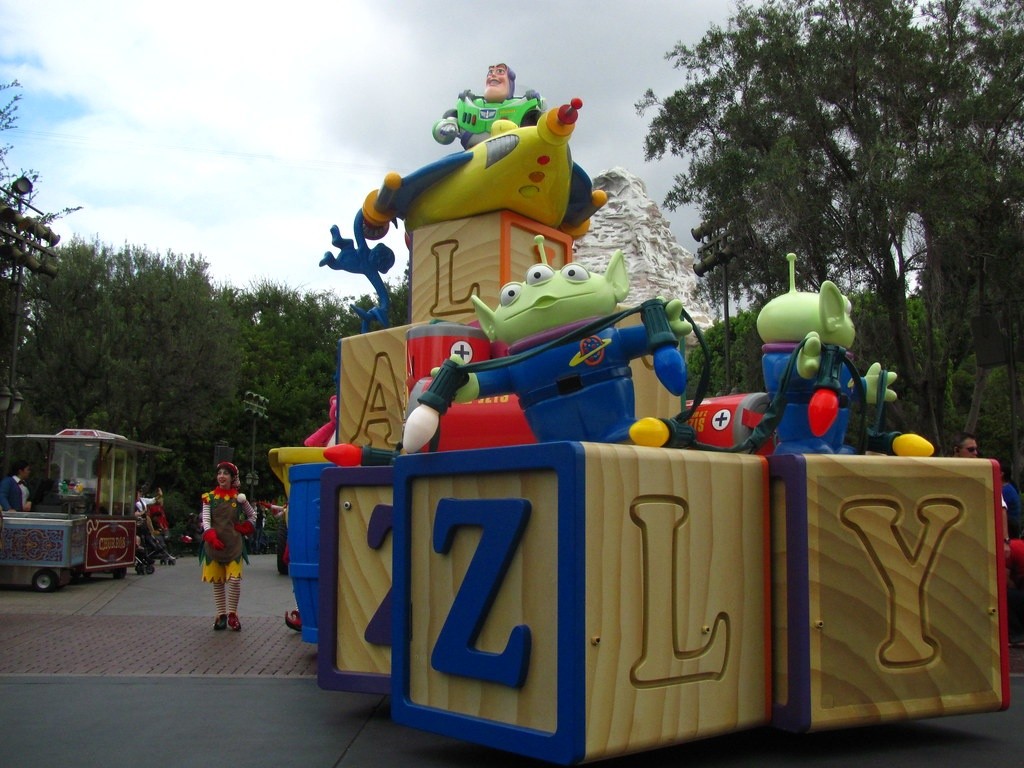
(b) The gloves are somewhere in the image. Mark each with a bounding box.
[258,502,271,508]
[203,527,225,552]
[235,521,254,536]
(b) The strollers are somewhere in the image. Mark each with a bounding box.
[133,518,177,575]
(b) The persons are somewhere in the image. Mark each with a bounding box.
[183,500,303,632]
[1,460,34,513]
[201,462,257,629]
[134,485,169,565]
[432,63,545,149]
[949,432,1024,646]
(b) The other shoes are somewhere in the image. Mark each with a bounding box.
[228,612,241,631]
[214,613,227,629]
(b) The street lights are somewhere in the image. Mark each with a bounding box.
[244,390,269,502]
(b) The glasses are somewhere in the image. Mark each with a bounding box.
[960,447,978,452]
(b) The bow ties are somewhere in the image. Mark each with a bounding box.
[19,481,25,484]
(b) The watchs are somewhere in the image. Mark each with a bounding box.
[1004,538,1010,544]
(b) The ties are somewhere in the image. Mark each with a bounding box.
[139,498,145,511]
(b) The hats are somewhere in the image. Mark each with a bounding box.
[216,462,241,490]
